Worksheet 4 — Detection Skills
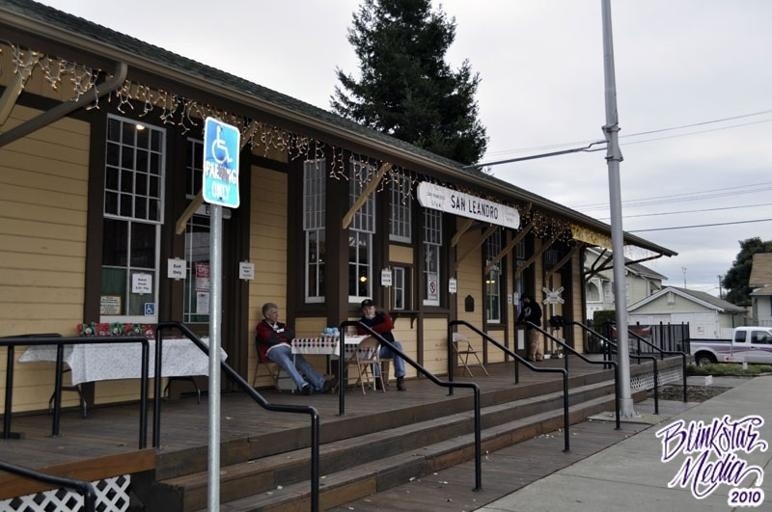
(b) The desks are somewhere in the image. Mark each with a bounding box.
[30,336,210,418]
[291,333,376,380]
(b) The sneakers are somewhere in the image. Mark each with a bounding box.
[303,385,314,395]
[322,377,339,392]
[396,377,407,390]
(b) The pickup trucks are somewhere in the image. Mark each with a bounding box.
[688,326,771,367]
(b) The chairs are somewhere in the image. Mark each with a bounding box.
[253,336,291,393]
[451,331,488,377]
[330,335,386,396]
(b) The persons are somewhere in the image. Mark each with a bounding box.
[355,299,407,391]
[256,302,336,395]
[515,292,545,363]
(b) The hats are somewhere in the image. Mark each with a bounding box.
[361,299,375,307]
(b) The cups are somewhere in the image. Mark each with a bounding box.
[323,328,339,334]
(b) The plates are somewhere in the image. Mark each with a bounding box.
[320,333,340,337]
